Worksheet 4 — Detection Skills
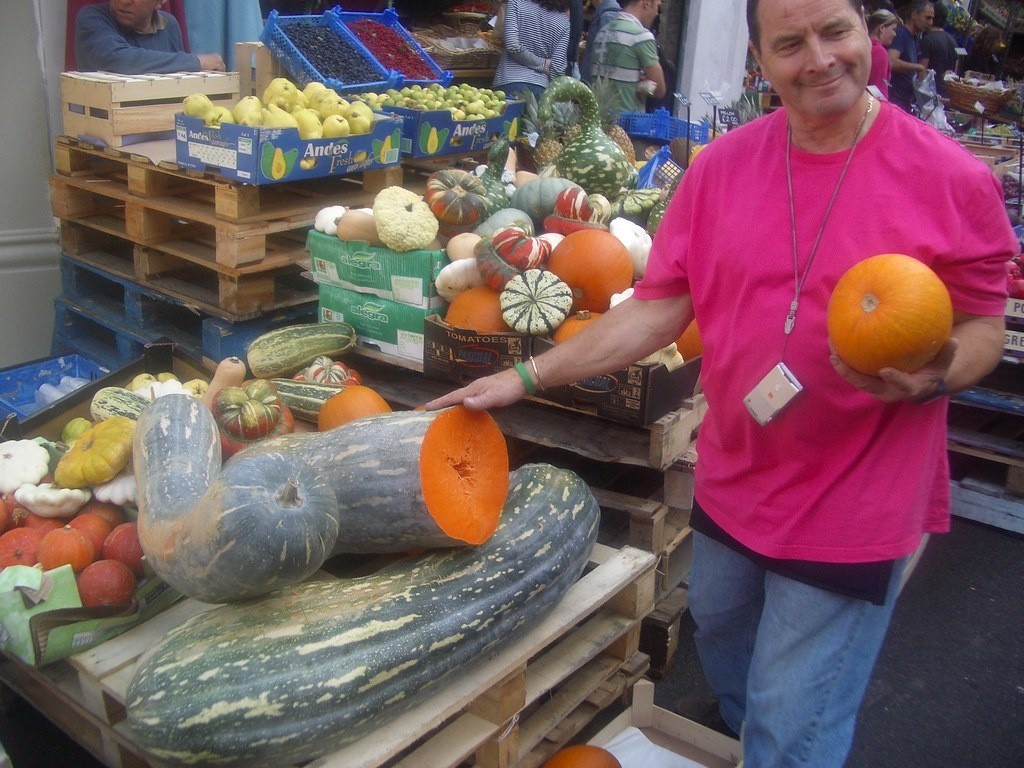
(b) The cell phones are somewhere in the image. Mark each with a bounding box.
[743,362,804,427]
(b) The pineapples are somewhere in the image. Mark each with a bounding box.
[514,72,636,172]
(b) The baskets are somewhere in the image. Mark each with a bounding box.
[332,5,453,88]
[607,107,709,143]
[941,71,1017,116]
[634,145,686,194]
[259,7,398,97]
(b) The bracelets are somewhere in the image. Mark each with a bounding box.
[529,356,544,391]
[513,362,536,394]
[913,381,945,406]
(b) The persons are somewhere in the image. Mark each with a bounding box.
[426,0,1021,768]
[887,0,934,114]
[918,2,959,98]
[566,0,666,113]
[957,26,1001,78]
[864,9,900,101]
[489,0,571,103]
[74,0,227,119]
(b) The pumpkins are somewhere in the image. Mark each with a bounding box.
[312,139,709,373]
[0,318,622,768]
[826,253,952,376]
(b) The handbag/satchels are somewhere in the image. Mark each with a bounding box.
[912,69,956,138]
[656,44,676,82]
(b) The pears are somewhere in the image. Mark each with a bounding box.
[182,78,374,139]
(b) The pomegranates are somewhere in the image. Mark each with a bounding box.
[1004,254,1024,300]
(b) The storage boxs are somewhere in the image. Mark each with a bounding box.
[533,336,702,427]
[978,0,1024,32]
[613,107,709,144]
[638,145,685,189]
[175,111,405,186]
[0,353,112,418]
[586,677,746,768]
[0,337,221,443]
[318,285,446,364]
[335,4,455,90]
[296,228,452,311]
[260,8,399,96]
[423,313,533,383]
[954,133,1024,174]
[380,96,527,159]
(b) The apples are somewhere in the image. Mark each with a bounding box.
[349,81,508,122]
